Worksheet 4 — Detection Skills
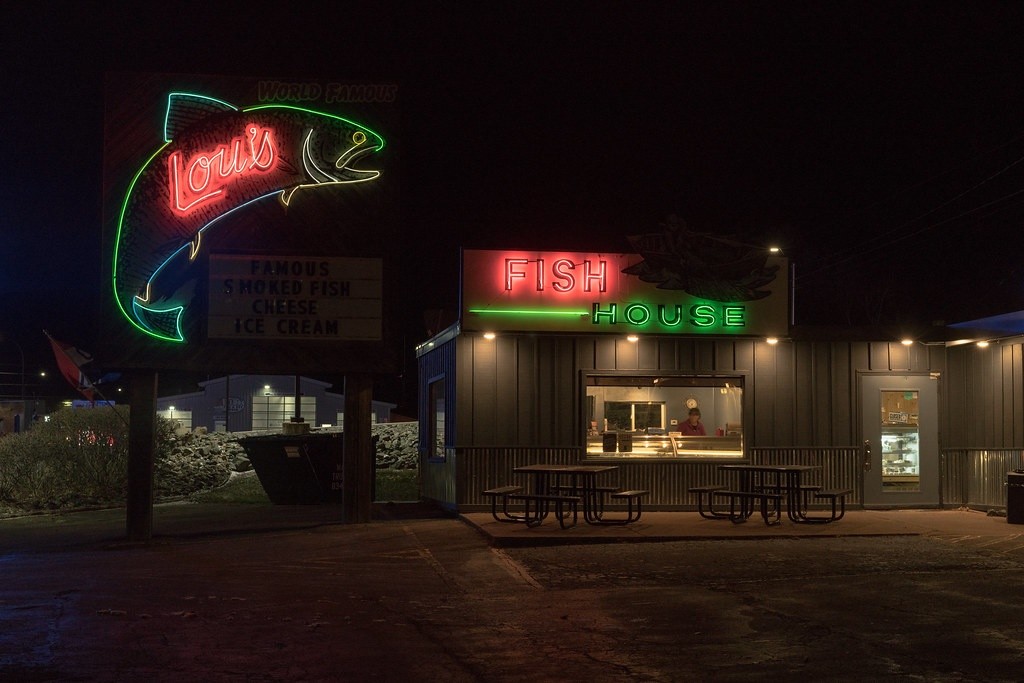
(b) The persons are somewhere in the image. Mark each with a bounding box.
[676,408,708,436]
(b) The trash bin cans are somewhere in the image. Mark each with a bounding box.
[1003,470,1024,524]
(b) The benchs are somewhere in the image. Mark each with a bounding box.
[482,485,651,530]
[689,483,854,526]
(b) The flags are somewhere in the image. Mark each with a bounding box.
[43,329,94,409]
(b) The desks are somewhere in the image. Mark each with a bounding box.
[717,464,823,518]
[511,463,620,521]
